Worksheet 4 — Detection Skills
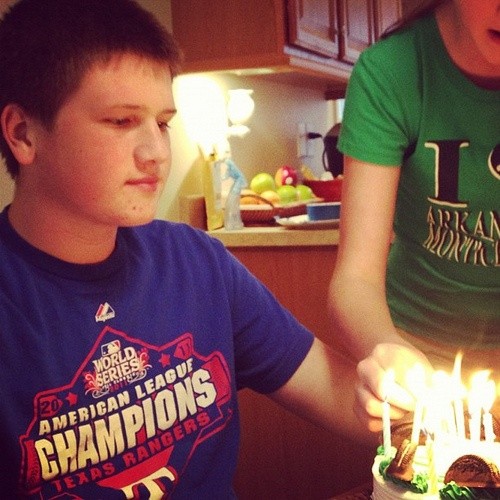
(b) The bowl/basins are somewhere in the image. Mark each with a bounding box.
[301,178,342,202]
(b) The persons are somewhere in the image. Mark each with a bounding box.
[0,0,425,500]
[327,0,500,433]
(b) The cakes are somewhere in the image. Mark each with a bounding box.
[371,407,500,500]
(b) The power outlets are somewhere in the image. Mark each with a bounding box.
[297,123,319,158]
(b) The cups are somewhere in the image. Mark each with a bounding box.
[306,202,341,220]
[178,195,207,231]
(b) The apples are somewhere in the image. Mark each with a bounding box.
[239,164,312,205]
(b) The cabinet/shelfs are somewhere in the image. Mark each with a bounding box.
[170,0,428,100]
[199,220,395,500]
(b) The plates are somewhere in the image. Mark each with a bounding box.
[276,216,340,230]
